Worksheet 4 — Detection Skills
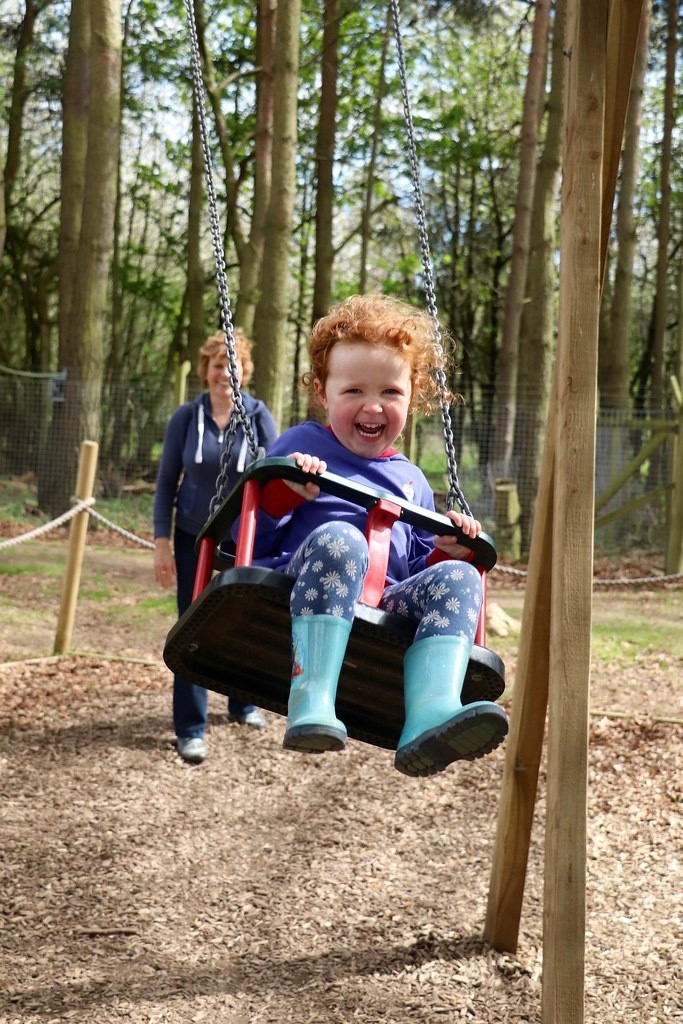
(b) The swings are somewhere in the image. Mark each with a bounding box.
[164,6,505,754]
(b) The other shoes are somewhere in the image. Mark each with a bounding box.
[230,710,266,729]
[178,737,206,759]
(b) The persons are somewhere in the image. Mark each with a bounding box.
[232,294,509,777]
[153,327,280,761]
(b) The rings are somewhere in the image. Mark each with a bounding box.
[162,571,167,575]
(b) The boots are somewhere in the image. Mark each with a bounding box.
[283,615,352,754]
[394,635,508,778]
